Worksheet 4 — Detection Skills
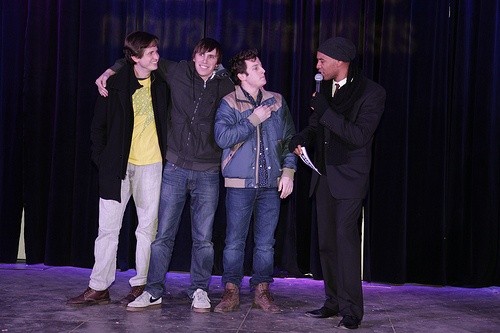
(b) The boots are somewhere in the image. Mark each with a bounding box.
[255,282,280,314]
[213,283,240,312]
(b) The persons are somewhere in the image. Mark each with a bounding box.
[289,35,387,329]
[214,50,299,314]
[61,31,172,306]
[95,38,235,312]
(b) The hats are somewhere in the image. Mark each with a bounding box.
[317,32,358,61]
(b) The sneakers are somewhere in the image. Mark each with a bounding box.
[189,289,211,313]
[127,291,162,312]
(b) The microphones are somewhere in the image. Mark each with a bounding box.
[314,73,323,93]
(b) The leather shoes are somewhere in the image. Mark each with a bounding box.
[338,313,361,329]
[120,287,144,304]
[304,308,334,317]
[66,290,110,307]
